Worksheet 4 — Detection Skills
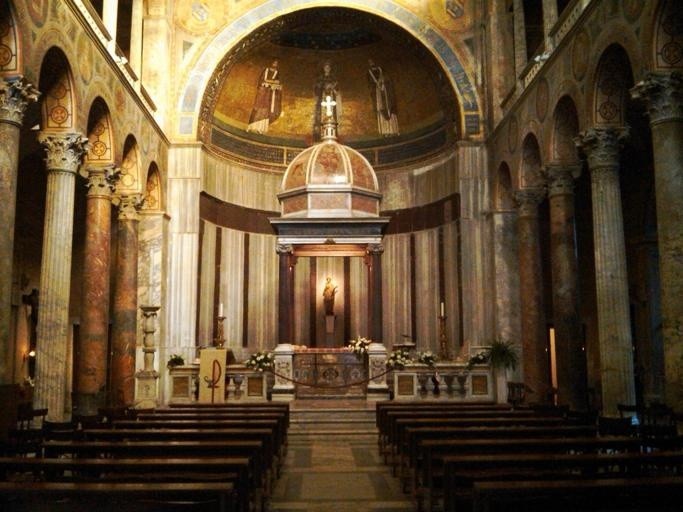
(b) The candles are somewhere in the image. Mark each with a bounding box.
[440,302,444,317]
[216,302,223,318]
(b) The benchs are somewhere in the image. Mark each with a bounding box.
[0,385,293,512]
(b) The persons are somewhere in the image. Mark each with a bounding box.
[365,59,403,138]
[311,58,344,141]
[322,275,337,315]
[244,55,284,136]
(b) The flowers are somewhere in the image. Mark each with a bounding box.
[346,332,373,356]
[464,346,490,373]
[164,349,188,367]
[382,347,415,372]
[244,349,275,375]
[418,349,440,371]
